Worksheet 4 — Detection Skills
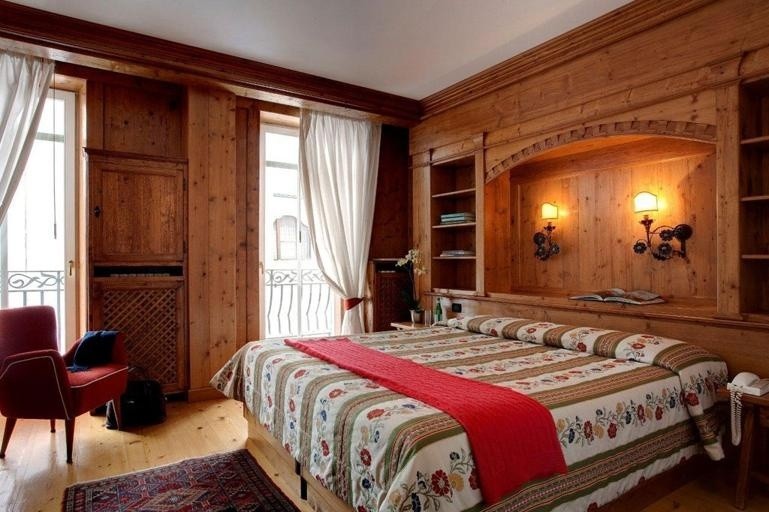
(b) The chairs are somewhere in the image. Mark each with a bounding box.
[0,305,129,465]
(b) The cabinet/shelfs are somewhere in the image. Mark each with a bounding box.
[363,257,414,332]
[717,76,768,321]
[425,135,486,296]
[75,82,191,398]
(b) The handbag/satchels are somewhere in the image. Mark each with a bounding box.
[104,365,168,429]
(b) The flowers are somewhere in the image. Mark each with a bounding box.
[400,247,429,308]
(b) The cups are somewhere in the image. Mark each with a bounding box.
[423,310,432,326]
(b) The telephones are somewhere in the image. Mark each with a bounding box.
[727,372,769,396]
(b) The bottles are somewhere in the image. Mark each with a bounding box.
[434,296,442,323]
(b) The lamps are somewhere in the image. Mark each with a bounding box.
[534,203,562,262]
[630,190,693,263]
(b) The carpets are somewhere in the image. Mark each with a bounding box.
[63,450,299,512]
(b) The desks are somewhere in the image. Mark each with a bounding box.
[716,378,767,511]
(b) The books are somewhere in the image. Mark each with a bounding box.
[442,251,475,255]
[440,254,472,256]
[440,220,474,225]
[440,211,475,218]
[568,287,665,306]
[441,216,475,222]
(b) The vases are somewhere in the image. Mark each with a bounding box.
[410,308,423,323]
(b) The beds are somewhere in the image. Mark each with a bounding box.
[241,317,725,511]
